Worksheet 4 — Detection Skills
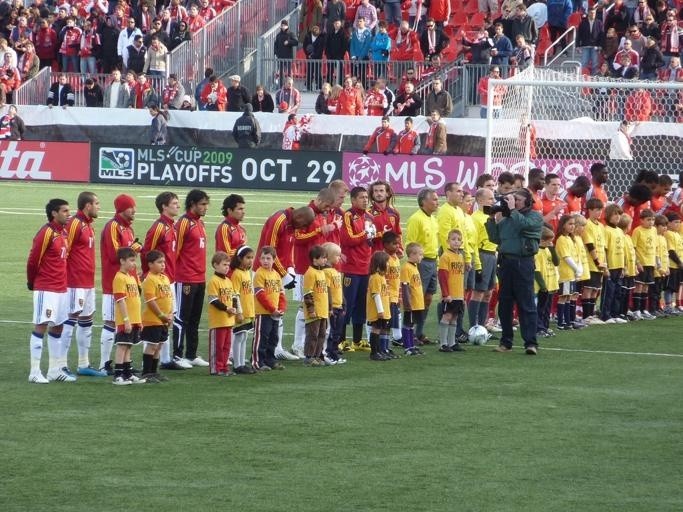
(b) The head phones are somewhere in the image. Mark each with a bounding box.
[521,188,533,208]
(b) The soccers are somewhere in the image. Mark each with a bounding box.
[469,325,488,345]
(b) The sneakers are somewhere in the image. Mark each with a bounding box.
[76,366,103,376]
[29,375,49,383]
[306,349,346,366]
[47,372,76,382]
[62,367,74,377]
[159,360,184,370]
[370,334,438,361]
[351,339,371,351]
[559,307,683,330]
[338,341,355,352]
[439,319,553,354]
[100,360,170,386]
[171,356,192,369]
[186,356,209,367]
[293,346,306,358]
[210,359,285,376]
[274,351,300,361]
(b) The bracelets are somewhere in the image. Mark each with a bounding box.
[120,316,128,322]
[156,312,165,319]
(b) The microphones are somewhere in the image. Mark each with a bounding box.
[518,206,528,211]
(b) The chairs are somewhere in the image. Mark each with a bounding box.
[293,0,583,75]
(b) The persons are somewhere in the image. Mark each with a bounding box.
[271,1,458,155]
[251,173,499,363]
[139,250,176,383]
[494,162,682,354]
[461,1,683,160]
[110,244,148,387]
[1,105,11,140]
[23,196,79,386]
[2,0,313,151]
[63,188,287,377]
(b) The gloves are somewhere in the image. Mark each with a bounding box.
[282,266,297,289]
[475,270,483,283]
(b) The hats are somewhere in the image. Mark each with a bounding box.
[114,194,136,214]
[228,74,241,82]
